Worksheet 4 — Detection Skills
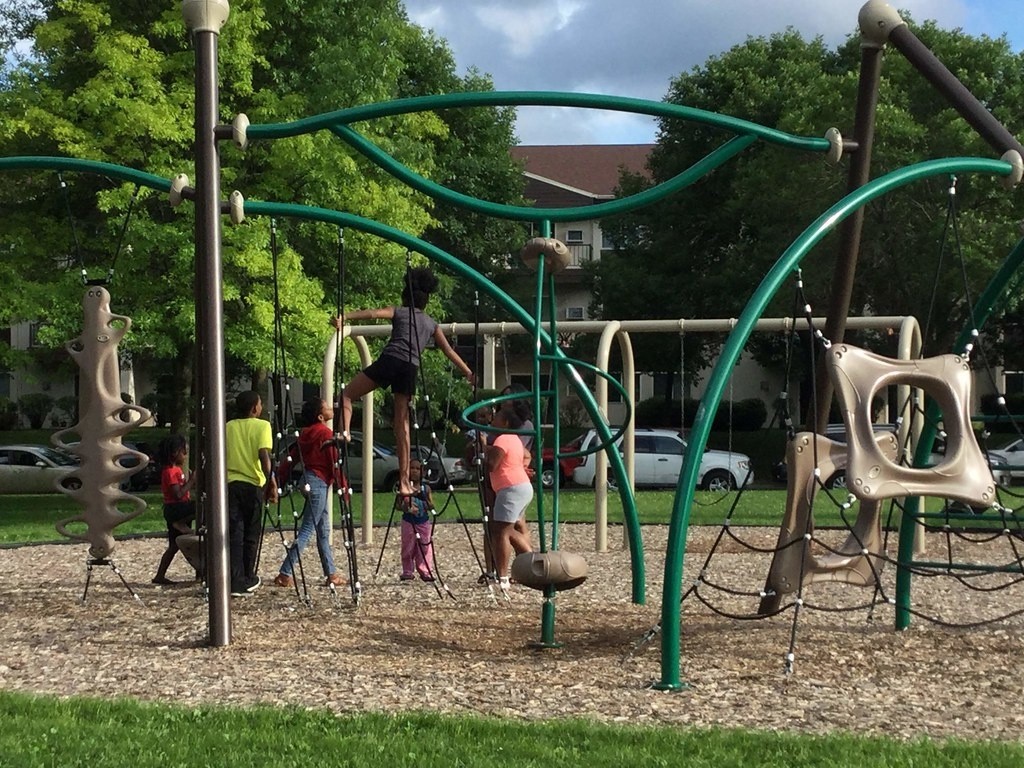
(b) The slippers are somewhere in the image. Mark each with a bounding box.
[320,438,355,451]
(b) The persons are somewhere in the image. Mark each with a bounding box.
[320,266,479,499]
[464,384,535,586]
[275,398,350,586]
[152,435,196,584]
[226,390,279,597]
[395,458,436,582]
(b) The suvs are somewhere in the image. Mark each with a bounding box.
[573,429,755,492]
[528,432,591,489]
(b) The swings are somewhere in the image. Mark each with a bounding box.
[408,338,456,486]
[679,331,734,506]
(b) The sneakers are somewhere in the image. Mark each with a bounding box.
[231,588,255,596]
[246,575,261,591]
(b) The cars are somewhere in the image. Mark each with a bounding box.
[273,428,411,492]
[0,443,172,494]
[771,424,1011,491]
[988,438,1024,478]
[394,444,466,491]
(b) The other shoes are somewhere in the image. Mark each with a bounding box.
[400,579,414,585]
[326,569,351,586]
[419,576,436,584]
[487,577,510,592]
[476,573,496,587]
[151,578,173,584]
[274,573,295,587]
